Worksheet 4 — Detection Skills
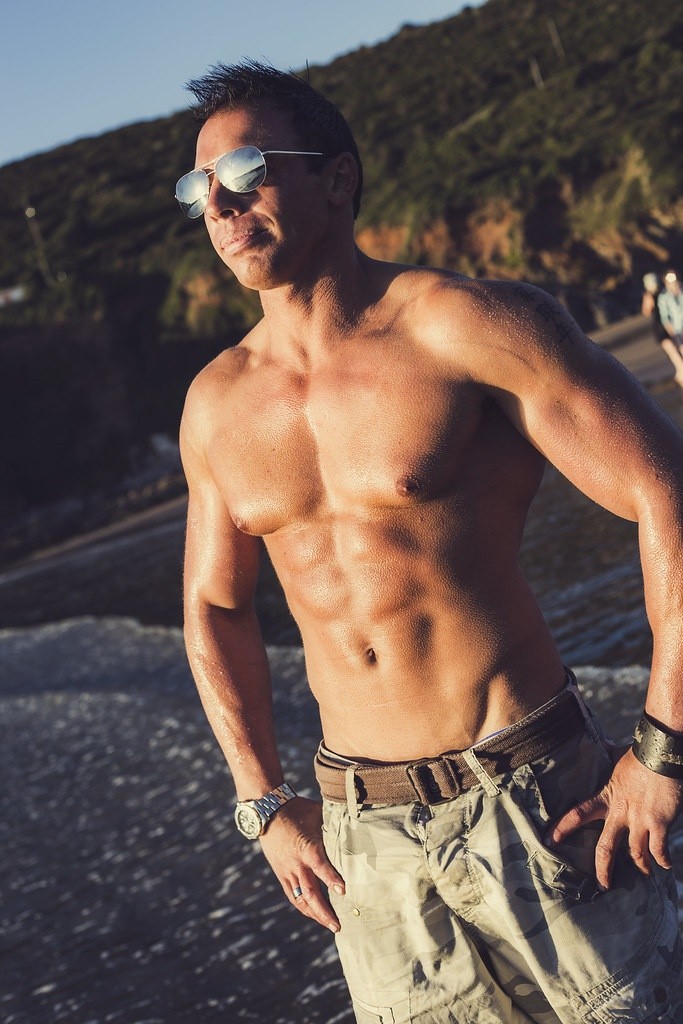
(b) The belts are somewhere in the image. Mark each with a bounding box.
[312,672,586,804]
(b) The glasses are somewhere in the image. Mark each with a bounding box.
[174,146,323,218]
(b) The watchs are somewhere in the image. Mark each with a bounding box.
[233,782,298,839]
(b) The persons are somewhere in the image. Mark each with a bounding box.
[658,267,683,360]
[175,58,683,1024]
[641,266,683,390]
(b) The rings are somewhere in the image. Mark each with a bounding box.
[292,884,305,899]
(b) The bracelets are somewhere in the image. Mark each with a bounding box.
[632,710,683,787]
